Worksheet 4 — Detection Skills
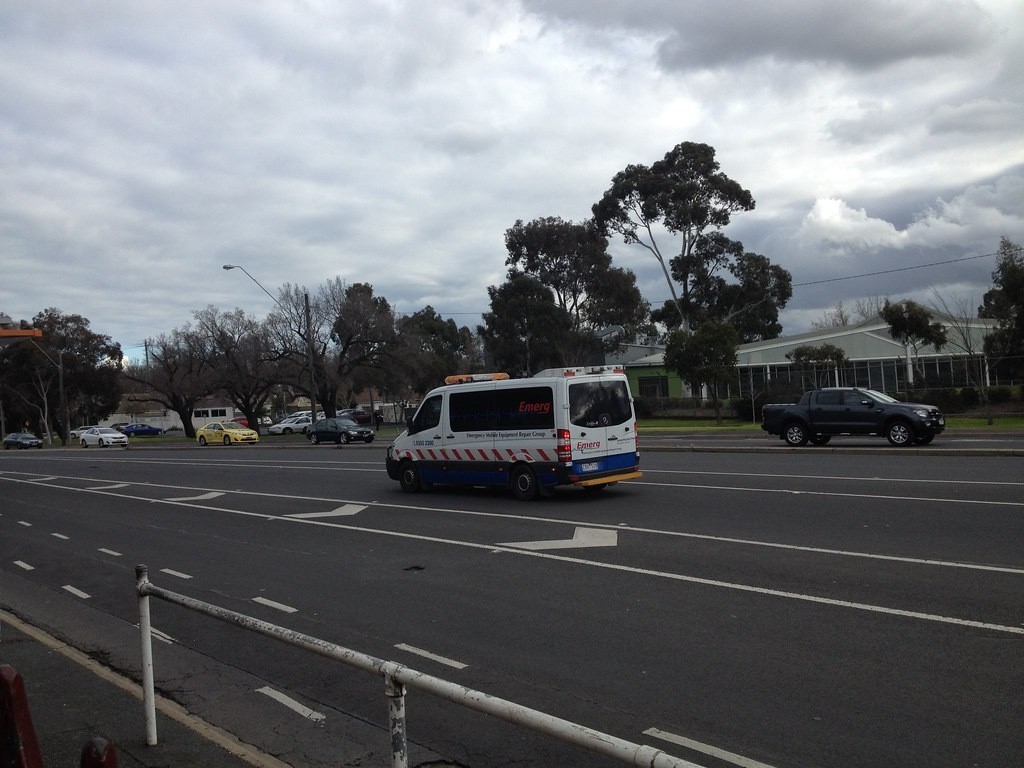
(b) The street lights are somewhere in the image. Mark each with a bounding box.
[223,264,317,423]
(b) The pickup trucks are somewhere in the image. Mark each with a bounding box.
[761,386,946,446]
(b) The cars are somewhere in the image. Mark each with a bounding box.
[231,416,274,425]
[79,428,129,448]
[196,422,260,446]
[292,410,326,420]
[307,417,375,445]
[70,426,103,439]
[4,433,43,450]
[111,423,129,432]
[268,417,313,434]
[336,409,372,423]
[121,423,165,436]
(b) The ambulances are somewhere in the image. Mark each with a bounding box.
[385,364,642,501]
